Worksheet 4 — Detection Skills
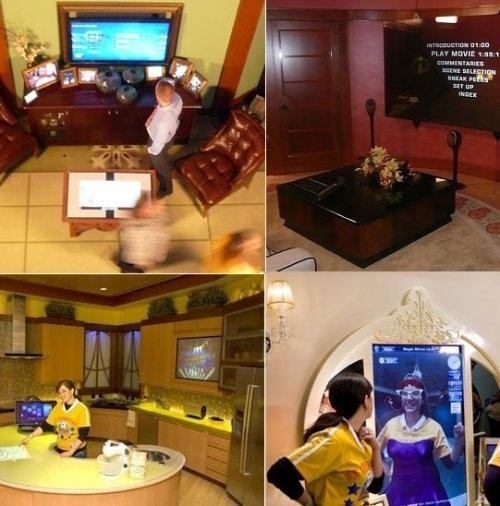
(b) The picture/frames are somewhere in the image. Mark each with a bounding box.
[181,69,208,99]
[58,64,79,90]
[77,66,99,84]
[21,57,60,92]
[146,64,166,83]
[168,57,194,85]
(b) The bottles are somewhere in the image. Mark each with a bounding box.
[96,66,145,104]
[120,398,126,406]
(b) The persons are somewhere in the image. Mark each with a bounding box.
[375,373,465,506]
[143,80,183,200]
[483,438,500,506]
[268,372,385,506]
[17,379,91,459]
[115,191,170,273]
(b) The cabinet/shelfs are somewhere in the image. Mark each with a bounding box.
[84,404,233,487]
[23,80,202,148]
[217,289,264,396]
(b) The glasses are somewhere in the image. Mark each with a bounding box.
[395,388,424,400]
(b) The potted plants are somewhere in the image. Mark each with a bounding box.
[42,301,77,321]
[186,286,230,313]
[146,297,177,321]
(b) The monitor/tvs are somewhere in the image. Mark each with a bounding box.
[16,400,57,426]
[67,13,172,66]
[383,14,500,131]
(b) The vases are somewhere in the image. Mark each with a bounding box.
[115,84,139,105]
[97,66,123,94]
[28,53,50,67]
[123,65,145,85]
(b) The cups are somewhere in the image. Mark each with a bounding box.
[155,392,170,410]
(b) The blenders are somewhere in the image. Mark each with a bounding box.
[97,439,130,480]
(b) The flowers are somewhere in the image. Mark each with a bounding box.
[4,23,53,66]
[355,143,405,189]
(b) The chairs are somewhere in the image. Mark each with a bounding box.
[0,96,41,177]
[265,242,318,272]
[169,108,266,219]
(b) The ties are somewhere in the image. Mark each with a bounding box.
[145,108,157,127]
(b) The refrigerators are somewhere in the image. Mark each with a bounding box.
[225,366,263,506]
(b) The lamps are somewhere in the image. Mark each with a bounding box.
[266,280,297,353]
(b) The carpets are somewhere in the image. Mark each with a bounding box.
[5,143,204,177]
[265,170,500,272]
